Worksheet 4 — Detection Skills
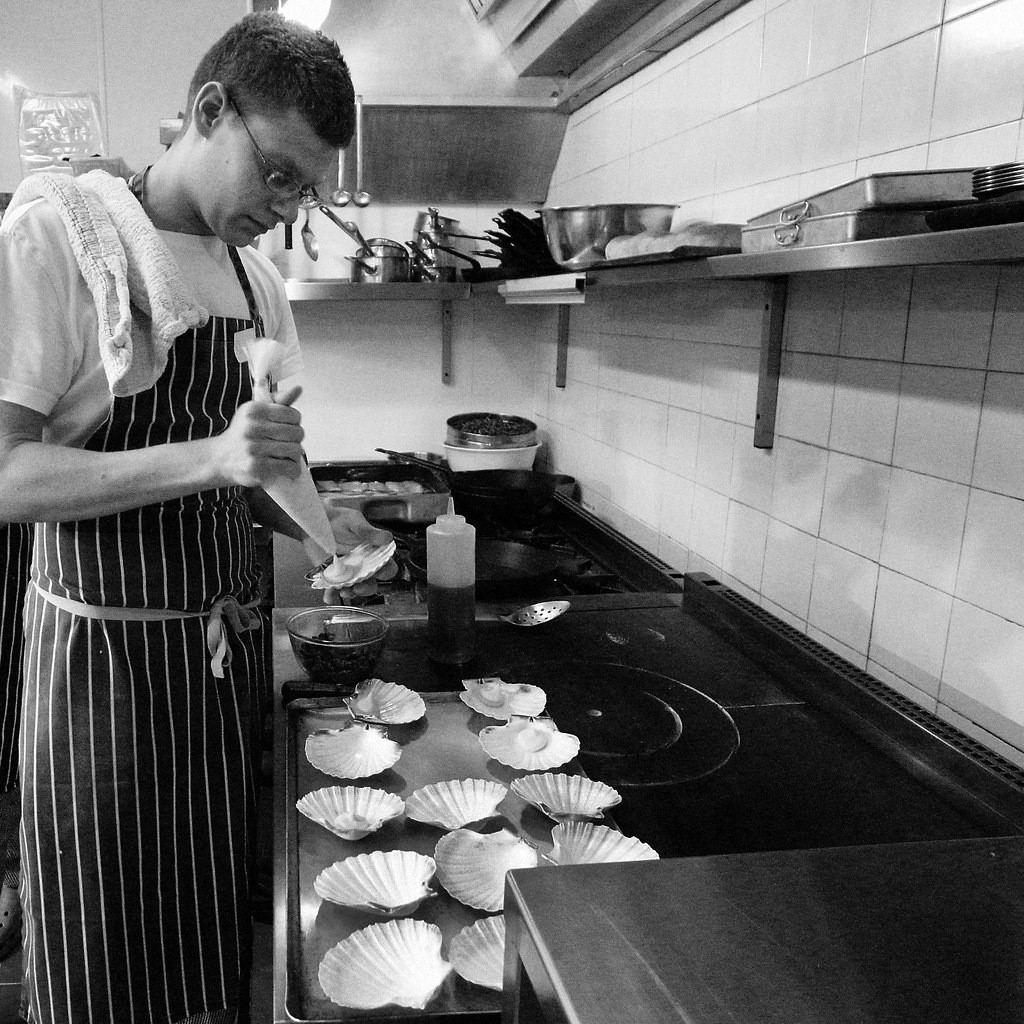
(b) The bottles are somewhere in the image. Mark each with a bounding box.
[426,496,478,662]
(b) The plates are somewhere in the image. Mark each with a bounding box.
[971,160,1024,201]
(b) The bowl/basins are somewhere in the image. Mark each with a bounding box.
[549,473,576,496]
[443,443,541,473]
[285,607,386,681]
[535,202,682,269]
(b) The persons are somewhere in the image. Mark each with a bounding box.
[0,10,400,1024]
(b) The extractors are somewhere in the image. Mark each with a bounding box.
[466,1,739,115]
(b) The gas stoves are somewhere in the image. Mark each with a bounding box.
[364,520,628,606]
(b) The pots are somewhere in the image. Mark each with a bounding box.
[319,203,568,285]
[372,524,561,588]
[375,447,555,518]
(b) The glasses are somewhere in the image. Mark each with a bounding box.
[226,93,324,209]
